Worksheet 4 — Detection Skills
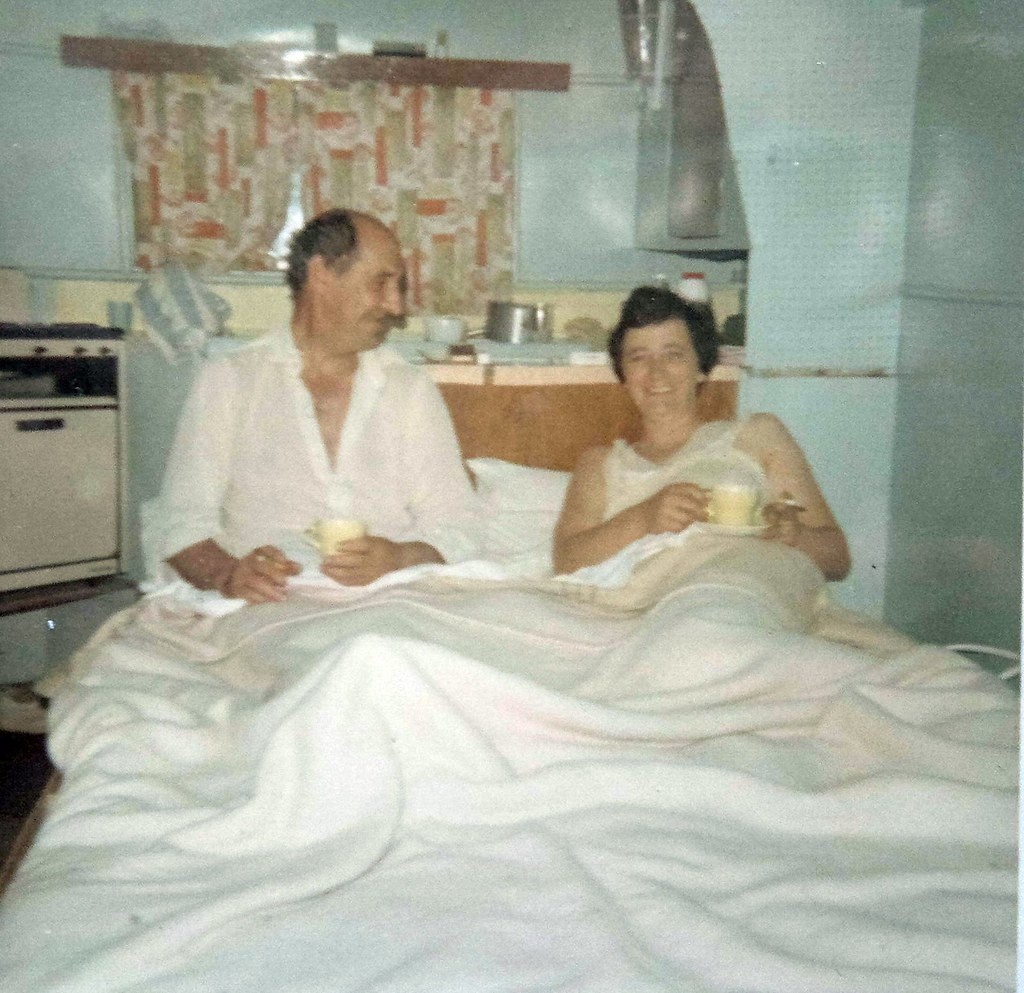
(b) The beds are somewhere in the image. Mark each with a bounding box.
[0,379,1021,993]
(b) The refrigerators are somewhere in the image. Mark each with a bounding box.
[1,337,131,591]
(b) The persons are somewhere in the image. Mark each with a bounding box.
[549,286,850,581]
[149,207,474,603]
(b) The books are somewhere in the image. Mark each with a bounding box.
[474,337,610,366]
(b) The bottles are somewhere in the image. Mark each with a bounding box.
[679,271,712,305]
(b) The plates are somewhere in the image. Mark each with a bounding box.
[696,517,775,537]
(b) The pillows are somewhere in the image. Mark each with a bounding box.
[465,455,573,514]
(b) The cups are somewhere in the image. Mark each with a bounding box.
[486,300,556,344]
[301,519,366,558]
[423,313,465,344]
[703,485,760,525]
[107,300,133,329]
